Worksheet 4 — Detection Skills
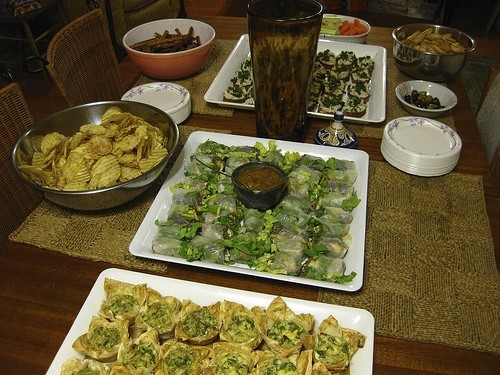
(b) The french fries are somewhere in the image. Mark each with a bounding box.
[399,28,465,53]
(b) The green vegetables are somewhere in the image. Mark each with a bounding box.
[154,139,361,284]
[223,50,374,119]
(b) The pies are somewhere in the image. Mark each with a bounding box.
[59,277,367,374]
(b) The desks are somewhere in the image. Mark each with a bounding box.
[0,15,500,375]
[0,0,67,92]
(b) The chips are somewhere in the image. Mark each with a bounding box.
[25,108,168,193]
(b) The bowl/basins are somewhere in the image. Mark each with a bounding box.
[122,18,216,80]
[11,100,180,211]
[231,161,289,212]
[391,23,478,83]
[319,13,372,43]
[394,79,458,119]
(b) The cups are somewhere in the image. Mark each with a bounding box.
[246,0,323,143]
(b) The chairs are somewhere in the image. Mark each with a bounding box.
[45,7,124,107]
[475,65,500,174]
[0,83,45,241]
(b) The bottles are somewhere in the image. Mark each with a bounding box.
[314,111,358,149]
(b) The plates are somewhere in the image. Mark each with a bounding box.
[121,81,192,125]
[380,115,462,178]
[128,131,370,292]
[45,267,375,375]
[203,32,387,124]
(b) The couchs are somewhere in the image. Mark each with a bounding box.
[59,0,187,64]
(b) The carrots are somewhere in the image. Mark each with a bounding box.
[339,19,368,36]
